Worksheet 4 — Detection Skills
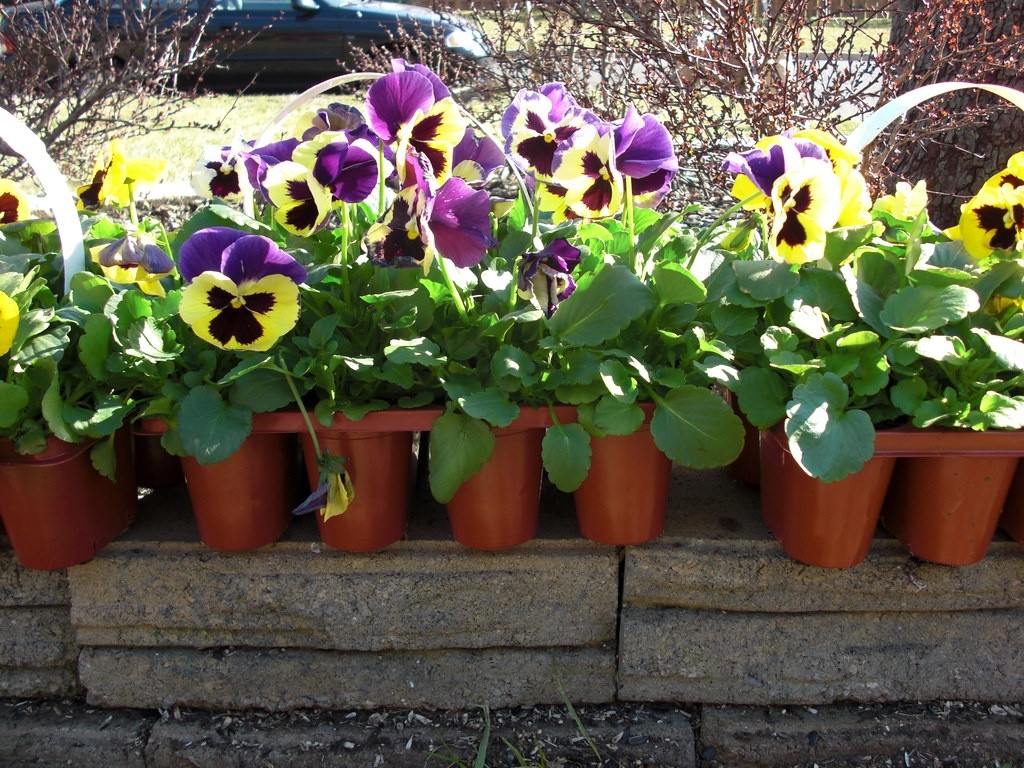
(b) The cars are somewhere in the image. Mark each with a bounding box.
[0,0,497,97]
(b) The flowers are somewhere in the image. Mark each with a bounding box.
[0,57,1024,527]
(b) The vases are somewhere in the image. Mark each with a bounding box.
[0,421,127,573]
[141,402,672,552]
[729,390,1024,569]
